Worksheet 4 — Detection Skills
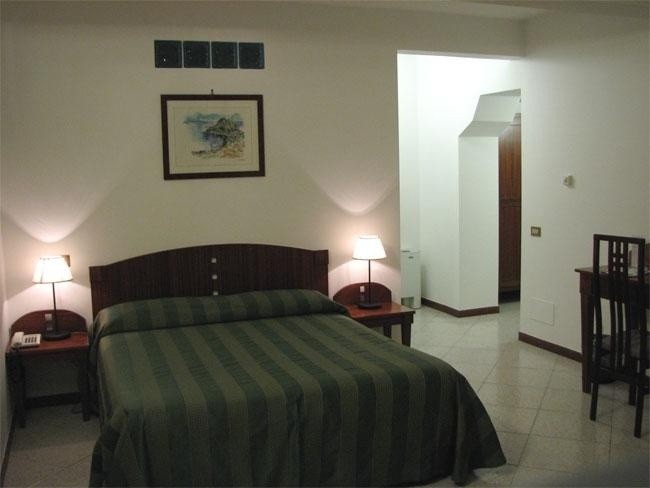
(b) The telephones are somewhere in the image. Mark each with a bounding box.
[11,331,42,349]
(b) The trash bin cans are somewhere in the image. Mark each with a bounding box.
[401,247,421,309]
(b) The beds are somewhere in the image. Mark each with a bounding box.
[85,243,508,488]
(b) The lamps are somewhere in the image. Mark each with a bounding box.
[352,235,386,309]
[32,253,74,341]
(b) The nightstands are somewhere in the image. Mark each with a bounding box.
[333,282,415,346]
[4,309,90,428]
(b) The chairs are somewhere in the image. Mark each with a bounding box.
[587,233,650,438]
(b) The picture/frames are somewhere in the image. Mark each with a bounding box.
[160,89,266,180]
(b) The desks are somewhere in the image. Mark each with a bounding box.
[575,264,650,405]
